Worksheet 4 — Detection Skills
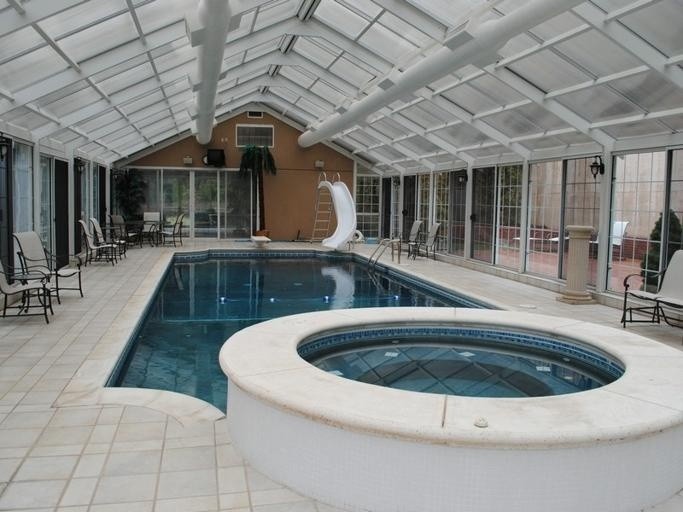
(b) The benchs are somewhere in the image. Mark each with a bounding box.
[620,250,683,328]
[512,236,568,258]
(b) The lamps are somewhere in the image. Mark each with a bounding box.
[0,131,10,161]
[111,172,118,183]
[458,169,468,185]
[75,162,84,176]
[589,154,605,178]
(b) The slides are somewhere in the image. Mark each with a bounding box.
[318,181,357,252]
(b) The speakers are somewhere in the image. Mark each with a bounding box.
[184,158,192,164]
[315,160,324,168]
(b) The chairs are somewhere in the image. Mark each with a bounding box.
[0,259,54,324]
[392,221,424,254]
[408,223,441,260]
[13,230,84,305]
[108,211,184,250]
[589,222,629,262]
[90,217,126,260]
[78,220,118,267]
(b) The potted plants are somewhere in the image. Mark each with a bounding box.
[239,144,277,243]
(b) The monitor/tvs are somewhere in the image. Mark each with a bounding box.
[208,149,224,165]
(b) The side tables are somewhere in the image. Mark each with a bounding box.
[11,274,54,315]
